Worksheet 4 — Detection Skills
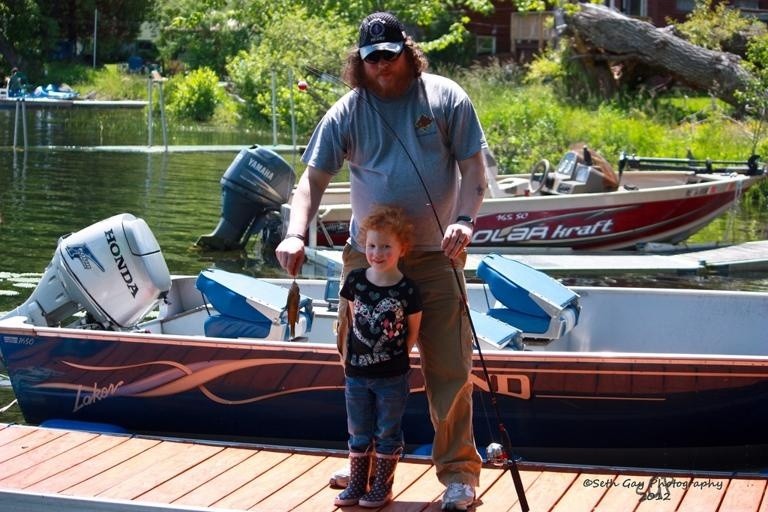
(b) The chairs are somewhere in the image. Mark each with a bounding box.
[481,143,529,198]
[194,269,313,341]
[470,253,582,352]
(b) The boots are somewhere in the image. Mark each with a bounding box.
[334,454,400,508]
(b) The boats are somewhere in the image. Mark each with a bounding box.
[1,81,80,101]
[0,212,766,437]
[0,93,149,109]
[192,144,767,253]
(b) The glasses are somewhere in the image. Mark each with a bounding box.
[357,11,407,60]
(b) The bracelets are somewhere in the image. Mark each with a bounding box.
[283,233,305,242]
[455,215,474,226]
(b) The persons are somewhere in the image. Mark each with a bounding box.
[333,204,425,508]
[271,10,492,512]
[7,68,74,100]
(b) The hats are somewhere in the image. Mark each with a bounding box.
[364,49,405,64]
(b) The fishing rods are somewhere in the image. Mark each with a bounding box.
[280,65,531,512]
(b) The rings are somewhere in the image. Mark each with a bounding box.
[457,239,463,244]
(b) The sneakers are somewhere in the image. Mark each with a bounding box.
[329,469,353,488]
[441,482,477,511]
